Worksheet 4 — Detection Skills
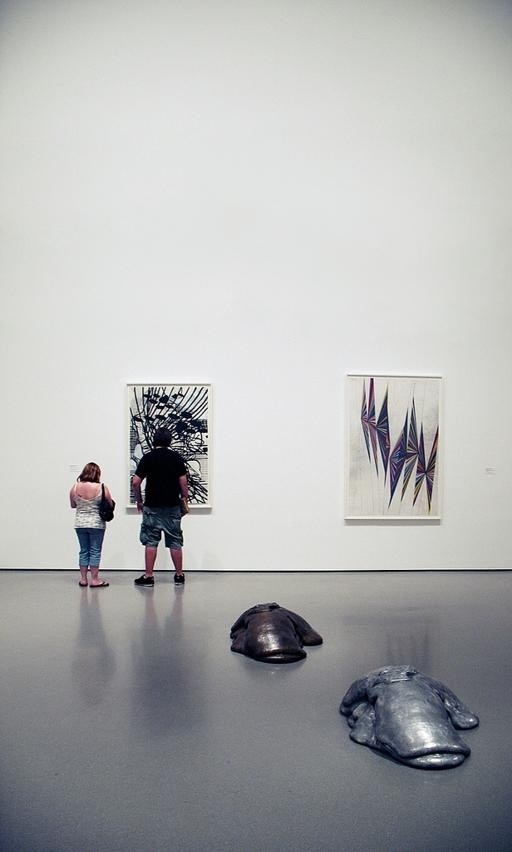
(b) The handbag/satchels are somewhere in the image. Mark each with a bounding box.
[99,499,115,522]
[180,499,189,515]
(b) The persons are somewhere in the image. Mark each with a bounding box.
[132,427,189,587]
[69,461,113,588]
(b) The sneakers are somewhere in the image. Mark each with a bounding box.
[135,574,154,587]
[174,573,184,584]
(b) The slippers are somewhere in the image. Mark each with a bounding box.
[79,581,88,586]
[90,581,109,587]
